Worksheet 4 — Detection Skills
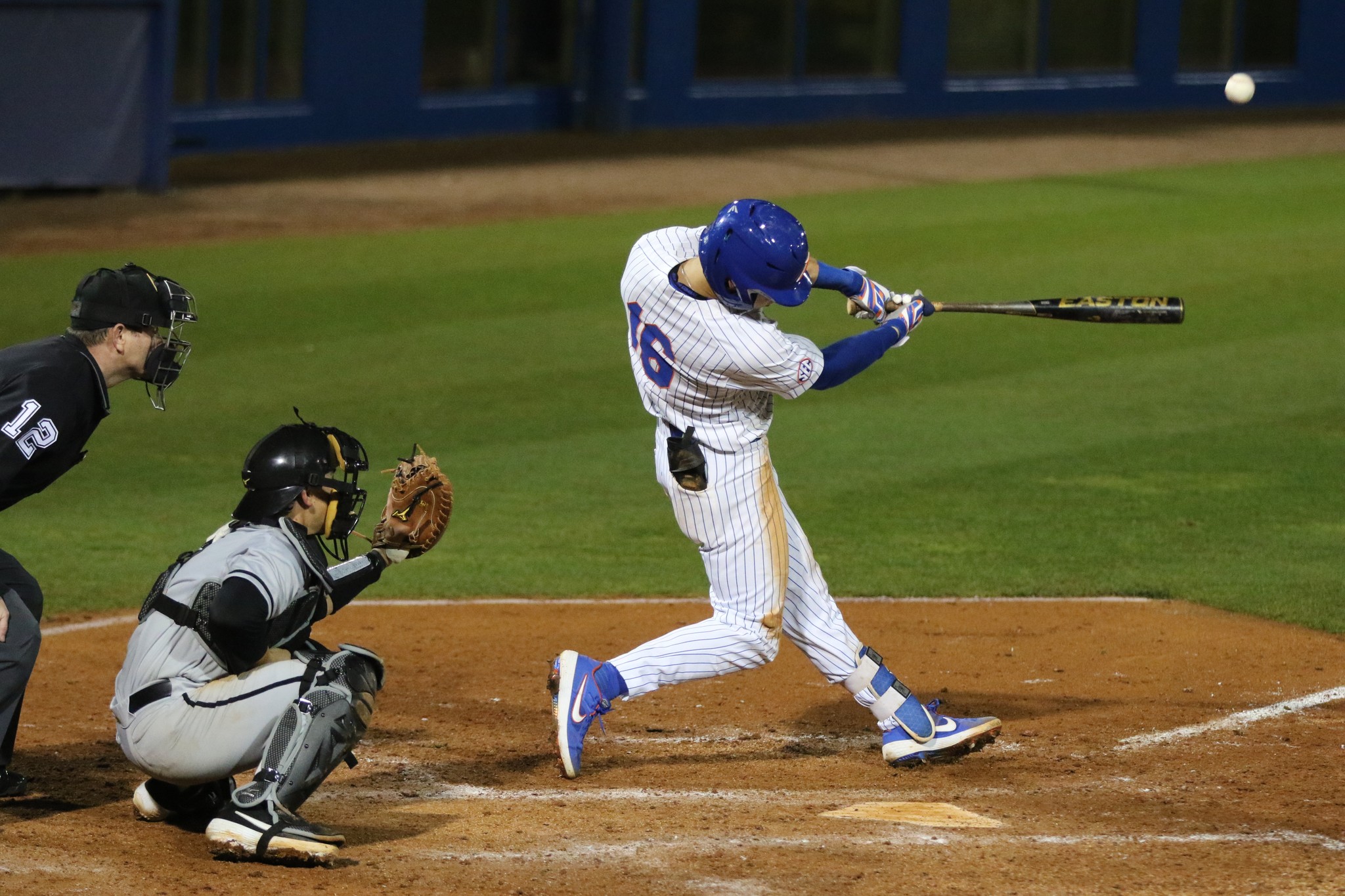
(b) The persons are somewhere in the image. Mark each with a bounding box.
[108,423,452,865]
[0,264,197,796]
[546,199,1001,780]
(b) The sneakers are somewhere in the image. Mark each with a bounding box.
[133,776,236,820]
[882,711,1001,767]
[549,650,611,778]
[205,800,339,868]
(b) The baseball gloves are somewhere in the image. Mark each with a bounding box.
[372,455,453,560]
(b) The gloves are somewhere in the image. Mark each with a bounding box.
[841,266,891,325]
[883,289,924,348]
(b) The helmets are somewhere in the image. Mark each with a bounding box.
[699,199,812,310]
[233,425,340,520]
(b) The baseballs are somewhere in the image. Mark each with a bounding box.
[1224,73,1256,104]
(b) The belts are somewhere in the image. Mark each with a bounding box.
[129,680,172,714]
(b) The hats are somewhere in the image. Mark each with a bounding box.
[70,265,172,333]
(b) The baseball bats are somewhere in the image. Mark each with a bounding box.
[847,295,1186,326]
